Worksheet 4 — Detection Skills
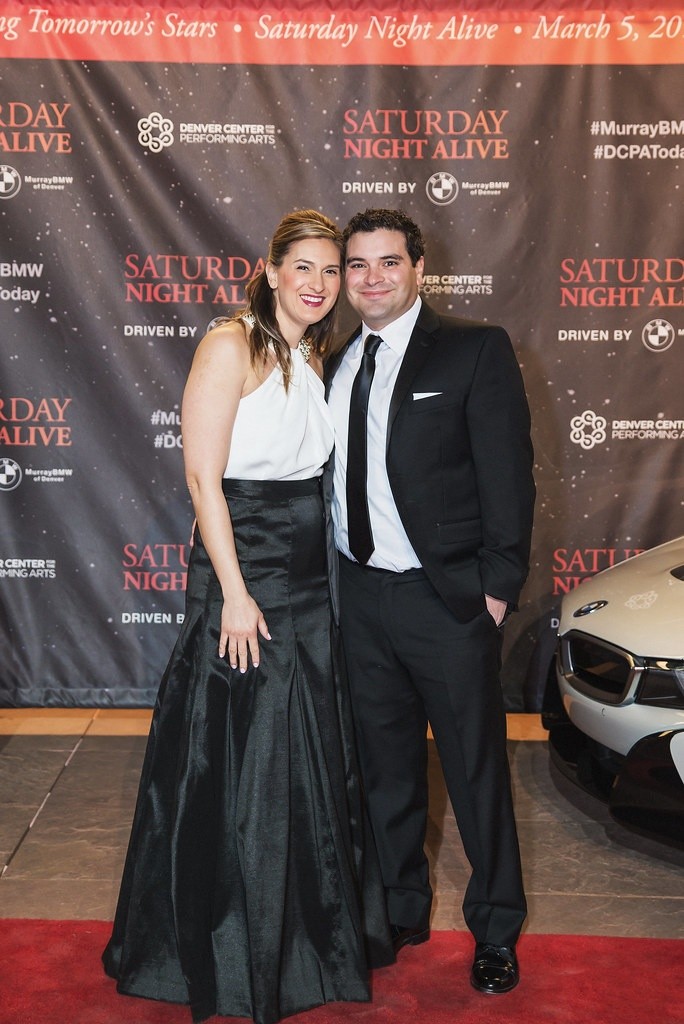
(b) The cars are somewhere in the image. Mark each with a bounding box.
[541,535,684,871]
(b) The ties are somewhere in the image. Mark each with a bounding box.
[345,334,384,565]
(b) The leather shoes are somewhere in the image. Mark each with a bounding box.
[470,937,519,993]
[391,925,431,955]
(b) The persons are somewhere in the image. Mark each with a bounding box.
[322,210,535,992]
[100,207,395,1024]
